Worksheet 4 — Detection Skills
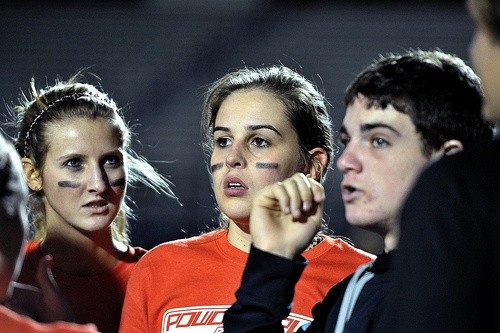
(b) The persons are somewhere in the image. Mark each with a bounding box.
[222,50,494,333]
[118,64,379,333]
[365,0,500,332]
[0,83,148,333]
[0,132,103,333]
